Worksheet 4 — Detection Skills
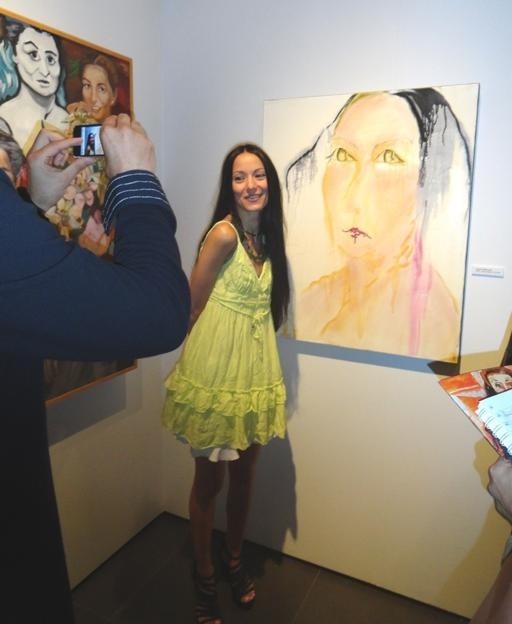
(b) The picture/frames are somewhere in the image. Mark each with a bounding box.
[1,7,140,408]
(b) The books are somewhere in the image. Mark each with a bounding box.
[437,365,512,459]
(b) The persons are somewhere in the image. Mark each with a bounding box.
[86,133,95,155]
[2,112,191,622]
[282,87,472,364]
[468,455,512,624]
[0,18,120,190]
[162,144,290,623]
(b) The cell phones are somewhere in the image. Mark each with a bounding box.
[73,124,105,158]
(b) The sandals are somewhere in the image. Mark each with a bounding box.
[189,542,257,624]
